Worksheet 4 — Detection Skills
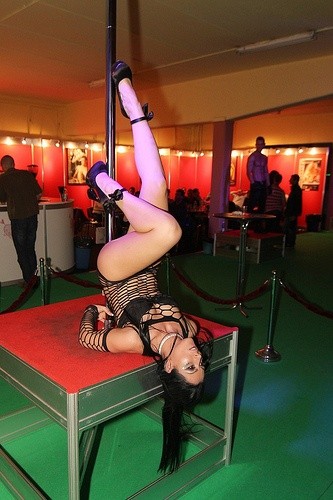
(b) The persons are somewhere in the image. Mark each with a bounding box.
[0,155,41,290]
[285,174,301,249]
[246,136,270,213]
[116,169,285,233]
[77,59,213,476]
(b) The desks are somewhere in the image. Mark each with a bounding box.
[213,211,276,318]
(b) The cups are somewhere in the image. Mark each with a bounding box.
[242,206,247,213]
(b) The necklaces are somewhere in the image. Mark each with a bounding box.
[157,333,183,355]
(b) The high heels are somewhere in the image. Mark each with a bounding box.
[86,161,107,203]
[114,60,138,117]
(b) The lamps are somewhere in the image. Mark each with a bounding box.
[275,148,280,153]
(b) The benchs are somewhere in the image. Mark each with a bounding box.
[213,229,286,265]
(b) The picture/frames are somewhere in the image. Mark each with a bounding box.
[299,158,322,189]
[65,147,88,184]
